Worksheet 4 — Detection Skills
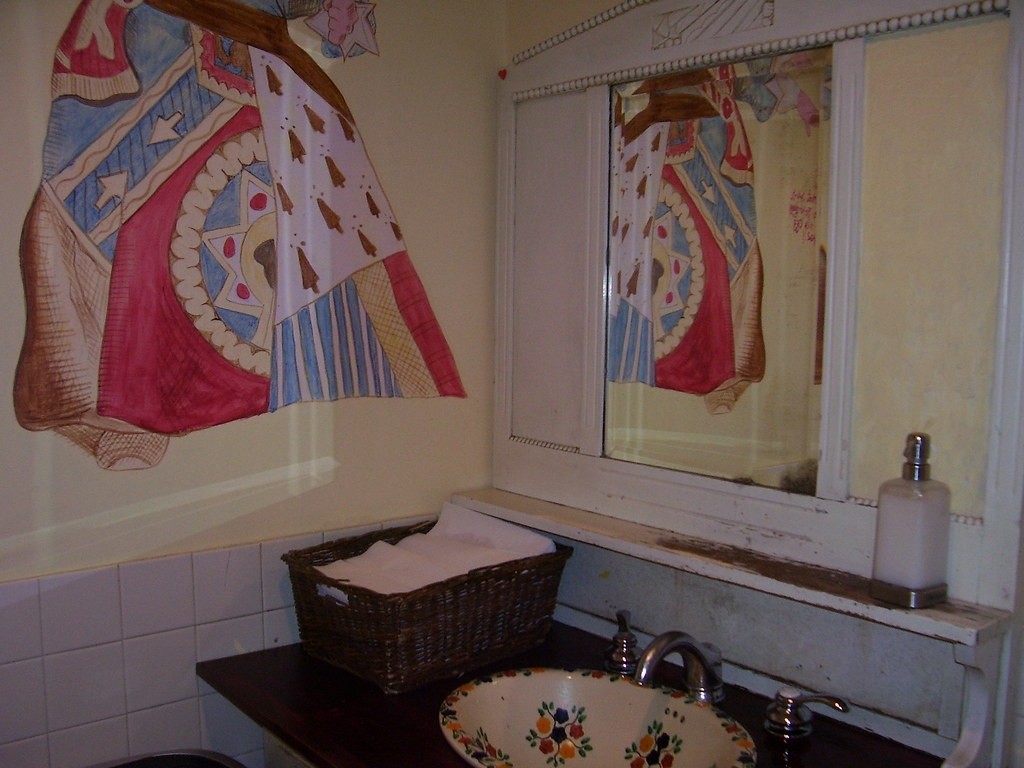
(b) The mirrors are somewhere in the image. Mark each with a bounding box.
[585,36,869,504]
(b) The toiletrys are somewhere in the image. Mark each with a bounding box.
[872,433,952,590]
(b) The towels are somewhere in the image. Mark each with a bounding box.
[310,500,556,594]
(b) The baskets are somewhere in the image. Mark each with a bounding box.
[280,517,574,705]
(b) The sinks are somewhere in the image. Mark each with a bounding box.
[435,665,758,768]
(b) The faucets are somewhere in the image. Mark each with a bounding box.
[629,630,726,702]
